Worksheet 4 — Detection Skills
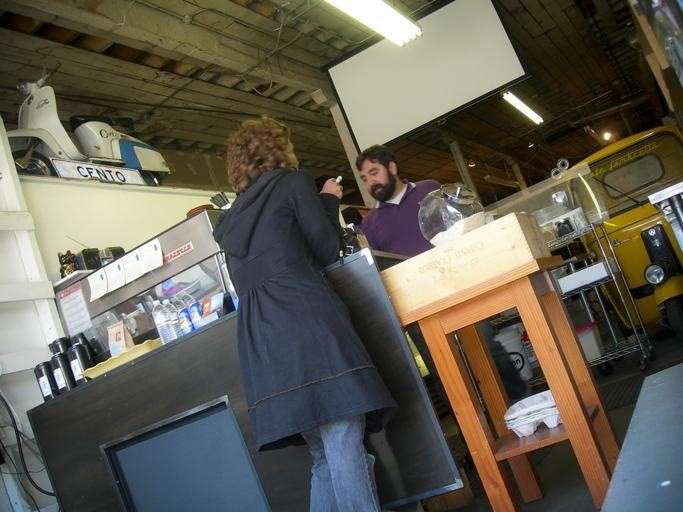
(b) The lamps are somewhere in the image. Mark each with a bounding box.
[324,0,423,49]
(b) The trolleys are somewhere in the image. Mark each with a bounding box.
[454,217,658,420]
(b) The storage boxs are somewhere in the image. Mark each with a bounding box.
[572,322,605,363]
[537,205,587,245]
[377,213,549,318]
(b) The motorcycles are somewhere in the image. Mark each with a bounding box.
[561,121,683,343]
[5,60,174,186]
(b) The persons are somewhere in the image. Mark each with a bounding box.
[208,116,399,511]
[354,144,526,436]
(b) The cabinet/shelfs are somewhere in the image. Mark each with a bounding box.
[55,207,237,390]
[397,254,620,510]
[473,206,654,396]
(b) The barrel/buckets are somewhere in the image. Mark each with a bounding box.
[504,323,538,364]
[494,329,533,382]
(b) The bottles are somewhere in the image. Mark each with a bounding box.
[152,291,206,345]
